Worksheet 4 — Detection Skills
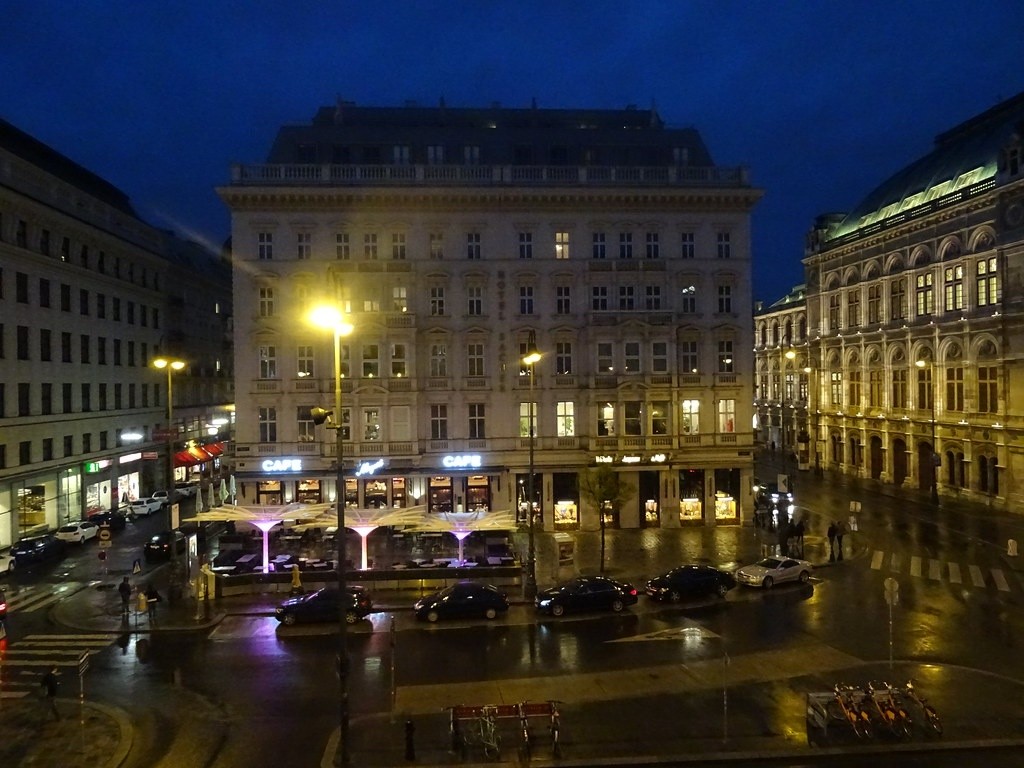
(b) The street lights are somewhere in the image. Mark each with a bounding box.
[310,304,355,583]
[523,354,546,595]
[787,351,820,477]
[916,360,940,506]
[154,357,187,599]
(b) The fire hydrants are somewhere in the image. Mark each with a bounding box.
[289,565,303,589]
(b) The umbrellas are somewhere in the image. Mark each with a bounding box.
[195,475,236,527]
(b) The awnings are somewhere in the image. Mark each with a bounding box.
[175,442,223,467]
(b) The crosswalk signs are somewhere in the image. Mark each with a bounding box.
[133,560,142,574]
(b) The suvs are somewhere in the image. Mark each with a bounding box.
[276,586,373,625]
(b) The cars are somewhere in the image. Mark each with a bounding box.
[413,581,510,622]
[535,576,639,617]
[88,481,198,531]
[0,554,16,619]
[736,554,813,589]
[10,534,66,567]
[55,521,100,545]
[144,527,192,562]
[645,563,736,605]
[754,478,795,518]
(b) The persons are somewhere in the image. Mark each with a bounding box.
[292,565,301,588]
[787,518,805,546]
[122,493,128,502]
[39,665,63,725]
[144,583,159,621]
[118,576,131,613]
[98,549,107,569]
[827,521,844,546]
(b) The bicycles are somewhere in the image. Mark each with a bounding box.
[828,680,945,740]
[440,698,565,752]
